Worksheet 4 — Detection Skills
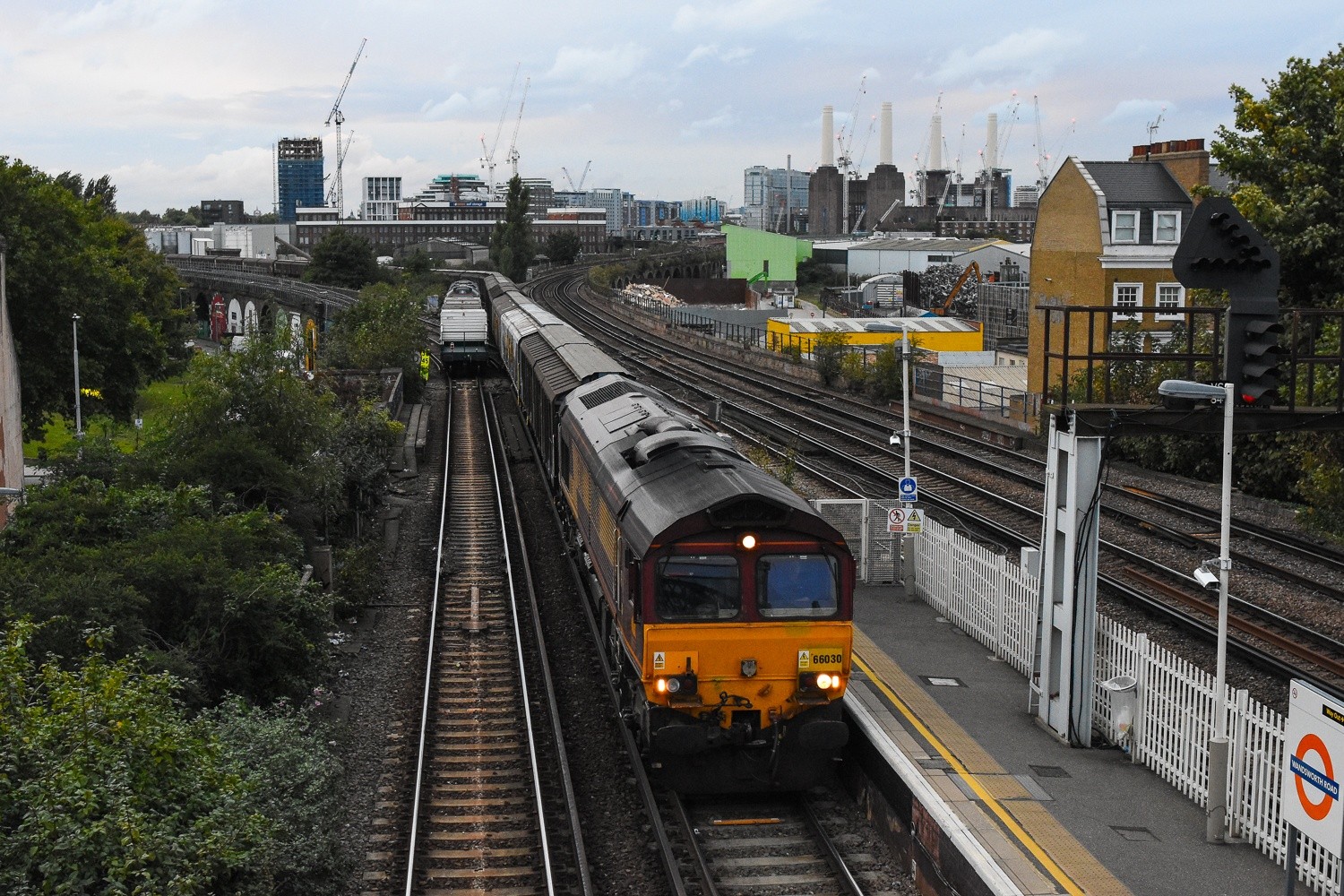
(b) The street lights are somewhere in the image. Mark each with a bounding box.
[1155,377,1235,845]
[865,322,917,605]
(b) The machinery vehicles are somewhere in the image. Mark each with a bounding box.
[928,260,982,316]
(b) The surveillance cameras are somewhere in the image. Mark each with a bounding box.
[889,436,901,446]
[1193,569,1222,590]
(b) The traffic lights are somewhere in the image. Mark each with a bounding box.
[1170,195,1279,292]
[1227,314,1290,412]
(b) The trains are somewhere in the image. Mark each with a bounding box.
[160,252,858,802]
[439,279,488,372]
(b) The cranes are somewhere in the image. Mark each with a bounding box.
[561,160,592,191]
[480,60,530,196]
[837,67,1167,236]
[324,37,368,221]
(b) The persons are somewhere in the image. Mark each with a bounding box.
[782,554,819,602]
